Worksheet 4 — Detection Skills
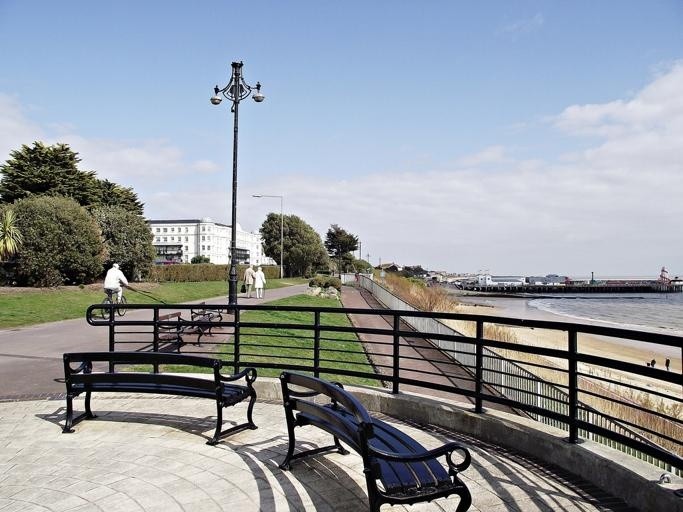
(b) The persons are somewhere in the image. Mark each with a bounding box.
[103,264,128,304]
[665,358,670,371]
[255,267,266,299]
[243,266,256,298]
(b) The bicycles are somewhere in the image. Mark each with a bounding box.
[100,283,128,320]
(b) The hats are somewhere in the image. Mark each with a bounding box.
[113,263,119,268]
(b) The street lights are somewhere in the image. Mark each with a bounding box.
[250,193,284,279]
[208,59,266,375]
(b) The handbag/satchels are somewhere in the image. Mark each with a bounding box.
[240,284,246,293]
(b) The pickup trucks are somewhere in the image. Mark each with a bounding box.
[309,270,335,287]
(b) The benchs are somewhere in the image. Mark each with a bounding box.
[153,311,204,352]
[279,369,472,512]
[191,301,224,336]
[61,351,257,444]
